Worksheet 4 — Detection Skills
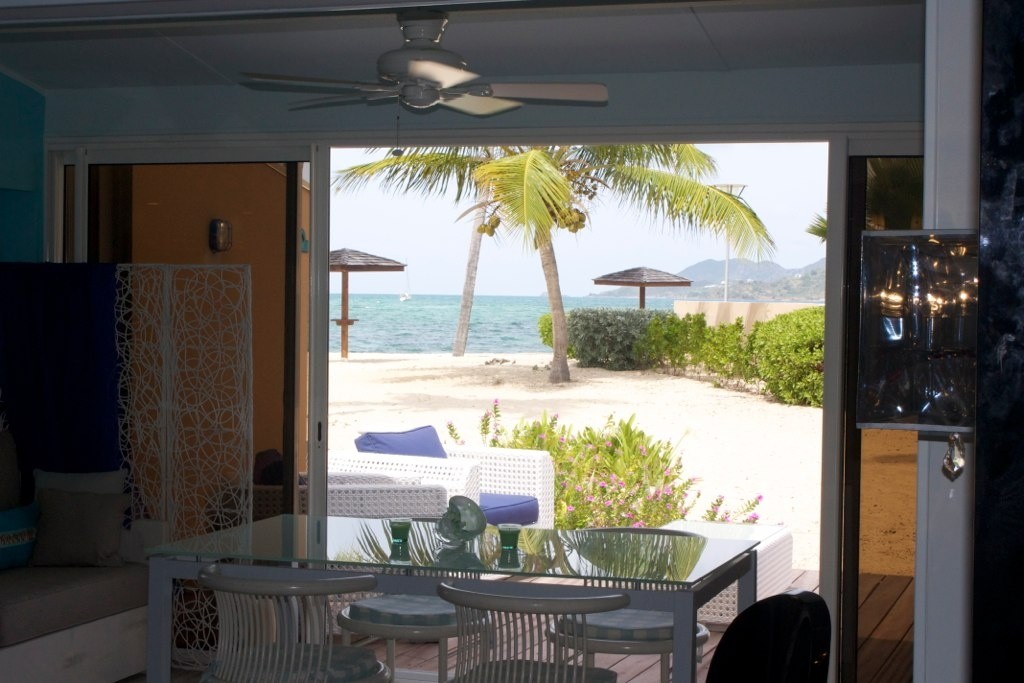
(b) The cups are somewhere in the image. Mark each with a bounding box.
[498,524,522,550]
[388,518,412,544]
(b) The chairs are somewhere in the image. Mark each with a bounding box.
[198,424,711,683]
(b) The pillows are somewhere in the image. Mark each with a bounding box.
[33,465,128,505]
[0,503,40,571]
[32,486,133,570]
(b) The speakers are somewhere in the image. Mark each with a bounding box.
[0,261,126,475]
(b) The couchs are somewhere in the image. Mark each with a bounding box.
[0,415,170,683]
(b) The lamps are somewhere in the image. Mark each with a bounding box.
[209,219,233,253]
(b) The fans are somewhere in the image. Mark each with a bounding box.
[235,6,610,120]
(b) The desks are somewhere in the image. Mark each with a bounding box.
[142,513,762,683]
[656,518,793,624]
